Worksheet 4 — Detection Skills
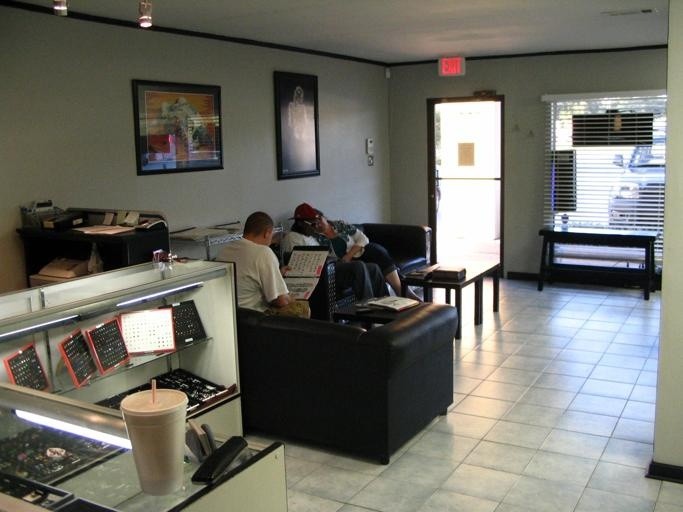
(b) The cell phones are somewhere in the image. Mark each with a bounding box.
[356,308,371,314]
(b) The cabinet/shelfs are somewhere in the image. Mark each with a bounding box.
[0,382,288,512]
[168,221,284,265]
[14,204,171,291]
[2,256,244,490]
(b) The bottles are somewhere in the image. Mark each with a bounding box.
[86,242,104,275]
[560,213,568,231]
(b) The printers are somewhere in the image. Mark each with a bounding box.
[29,256,90,288]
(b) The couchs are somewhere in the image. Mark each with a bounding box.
[270,218,435,322]
[235,301,461,467]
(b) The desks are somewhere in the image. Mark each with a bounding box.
[536,225,659,303]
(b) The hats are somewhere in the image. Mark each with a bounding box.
[294,203,320,220]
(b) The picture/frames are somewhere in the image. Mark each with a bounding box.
[3,340,47,394]
[84,318,129,376]
[271,69,321,182]
[116,306,174,353]
[130,77,225,176]
[55,328,101,389]
[158,299,209,343]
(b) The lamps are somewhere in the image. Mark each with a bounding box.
[52,0,69,16]
[136,0,153,28]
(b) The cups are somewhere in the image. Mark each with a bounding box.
[119,389,189,498]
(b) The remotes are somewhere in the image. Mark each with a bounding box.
[191,435,248,485]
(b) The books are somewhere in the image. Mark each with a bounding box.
[188,417,213,457]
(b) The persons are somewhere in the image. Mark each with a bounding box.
[218,211,311,317]
[302,207,426,305]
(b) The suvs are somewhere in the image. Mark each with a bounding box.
[606,131,666,232]
